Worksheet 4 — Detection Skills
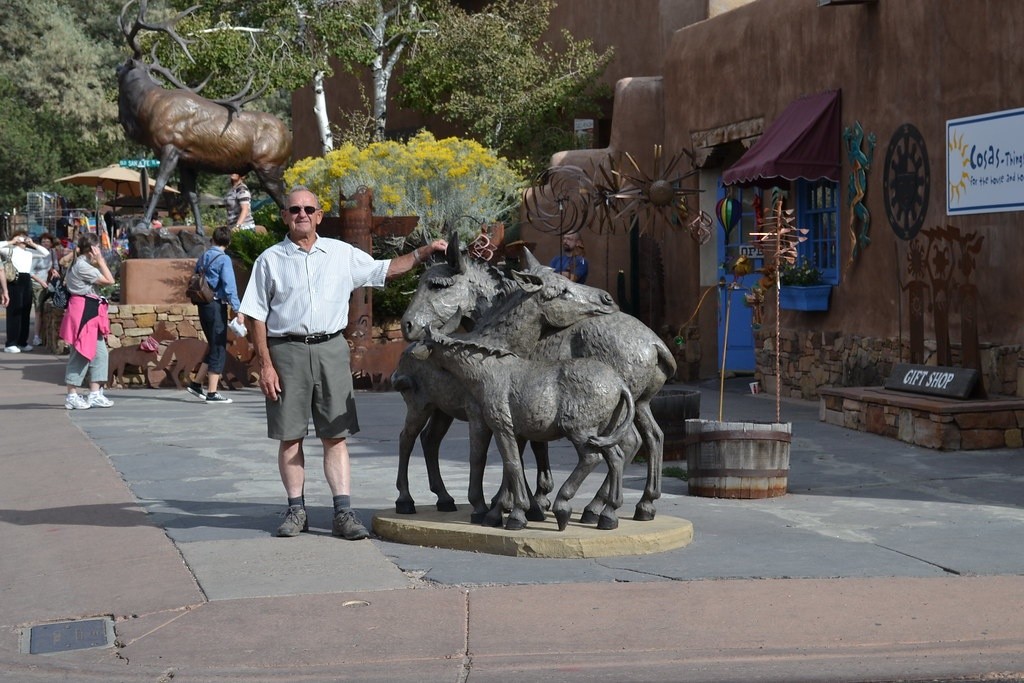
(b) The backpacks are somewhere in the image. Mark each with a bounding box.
[184,254,221,305]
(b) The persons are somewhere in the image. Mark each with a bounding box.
[0,228,79,353]
[549,229,589,283]
[59,233,115,410]
[152,210,162,228]
[187,226,245,404]
[225,173,257,235]
[239,185,448,540]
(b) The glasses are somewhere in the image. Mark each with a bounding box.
[283,205,320,216]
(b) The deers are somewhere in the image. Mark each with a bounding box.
[116,0,292,233]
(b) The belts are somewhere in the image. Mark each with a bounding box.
[278,331,342,345]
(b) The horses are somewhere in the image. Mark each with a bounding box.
[391,231,678,534]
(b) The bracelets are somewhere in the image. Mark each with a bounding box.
[413,249,421,263]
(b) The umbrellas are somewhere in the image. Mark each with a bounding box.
[54,164,182,244]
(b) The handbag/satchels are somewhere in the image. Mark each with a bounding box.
[53,282,70,309]
[5,246,19,283]
[47,264,58,294]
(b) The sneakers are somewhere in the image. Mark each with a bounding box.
[3,344,21,354]
[18,343,34,352]
[331,507,370,541]
[87,386,114,407]
[276,504,308,537]
[63,394,91,410]
[187,382,208,401]
[205,391,233,404]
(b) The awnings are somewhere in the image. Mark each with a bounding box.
[721,88,841,187]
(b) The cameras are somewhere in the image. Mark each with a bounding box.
[19,237,26,242]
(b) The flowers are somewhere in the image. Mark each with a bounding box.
[780,259,823,284]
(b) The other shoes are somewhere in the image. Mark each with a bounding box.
[33,336,42,346]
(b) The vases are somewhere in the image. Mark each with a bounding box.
[779,285,832,311]
